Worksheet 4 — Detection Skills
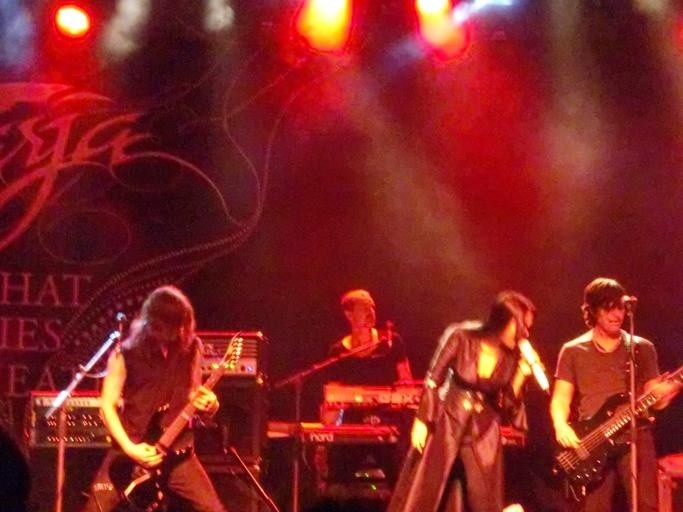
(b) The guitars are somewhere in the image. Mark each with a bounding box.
[546,369,682,483]
[108,330,244,512]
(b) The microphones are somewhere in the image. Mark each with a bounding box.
[116,313,127,353]
[385,320,394,347]
[622,295,638,315]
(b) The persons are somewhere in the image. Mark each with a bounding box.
[86,285,226,510]
[388,292,547,512]
[548,277,678,510]
[322,288,415,424]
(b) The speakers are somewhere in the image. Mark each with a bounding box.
[164,463,261,512]
[194,376,267,466]
